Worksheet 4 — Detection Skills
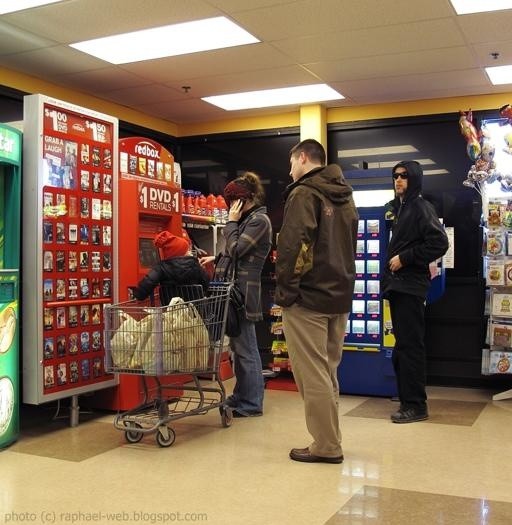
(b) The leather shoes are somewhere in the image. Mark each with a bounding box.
[219,405,246,419]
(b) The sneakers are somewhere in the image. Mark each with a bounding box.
[288,447,345,465]
[389,406,430,424]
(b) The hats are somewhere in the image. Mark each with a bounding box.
[153,231,190,262]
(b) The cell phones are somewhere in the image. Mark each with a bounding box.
[237,197,247,212]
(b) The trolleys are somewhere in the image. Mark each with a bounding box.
[105,281,233,448]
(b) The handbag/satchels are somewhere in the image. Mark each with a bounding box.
[209,244,242,338]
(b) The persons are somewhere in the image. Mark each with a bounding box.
[102,175,112,194]
[219,171,273,419]
[65,145,76,190]
[45,288,52,297]
[273,138,360,464]
[379,159,449,424]
[46,157,59,186]
[126,229,211,320]
[60,370,66,386]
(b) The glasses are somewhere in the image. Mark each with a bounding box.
[391,170,410,180]
